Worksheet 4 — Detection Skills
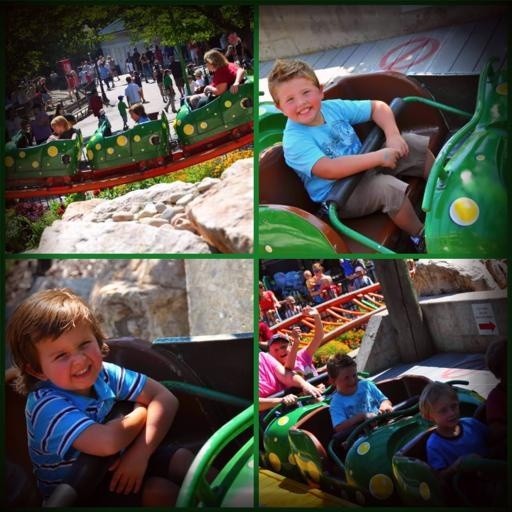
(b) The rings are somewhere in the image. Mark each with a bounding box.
[129,478,135,482]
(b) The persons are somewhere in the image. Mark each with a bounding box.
[7,287,218,507]
[258,352,322,430]
[269,59,435,253]
[270,305,324,380]
[259,258,415,351]
[6,31,254,144]
[328,351,393,451]
[485,337,507,434]
[419,381,504,505]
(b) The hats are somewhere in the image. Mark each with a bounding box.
[313,262,322,271]
[286,296,296,305]
[267,333,289,345]
[355,266,363,272]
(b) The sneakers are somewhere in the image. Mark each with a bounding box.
[408,224,427,253]
